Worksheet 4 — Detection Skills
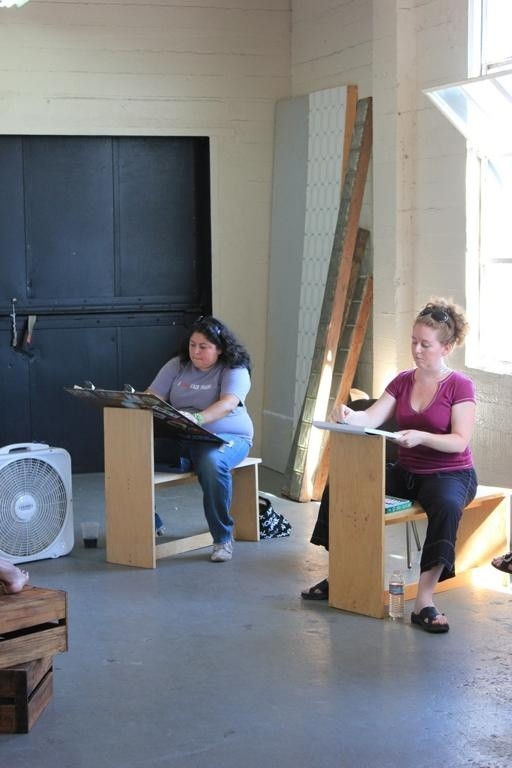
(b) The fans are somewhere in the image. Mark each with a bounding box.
[1,442,76,569]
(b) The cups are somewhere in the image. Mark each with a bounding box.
[79,520,100,549]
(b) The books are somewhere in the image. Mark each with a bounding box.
[312,420,399,445]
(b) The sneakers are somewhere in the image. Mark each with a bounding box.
[210,535,233,562]
[156,525,168,538]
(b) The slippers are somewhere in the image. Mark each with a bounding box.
[302,578,329,600]
[412,607,449,633]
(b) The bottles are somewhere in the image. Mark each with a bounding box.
[387,568,407,621]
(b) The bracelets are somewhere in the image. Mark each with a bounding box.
[192,411,206,427]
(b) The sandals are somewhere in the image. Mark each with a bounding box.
[492,552,512,574]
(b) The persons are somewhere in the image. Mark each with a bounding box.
[491,550,511,577]
[144,315,255,562]
[297,295,478,635]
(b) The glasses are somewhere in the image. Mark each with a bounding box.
[420,307,453,329]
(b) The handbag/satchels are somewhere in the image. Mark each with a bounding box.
[259,495,294,539]
